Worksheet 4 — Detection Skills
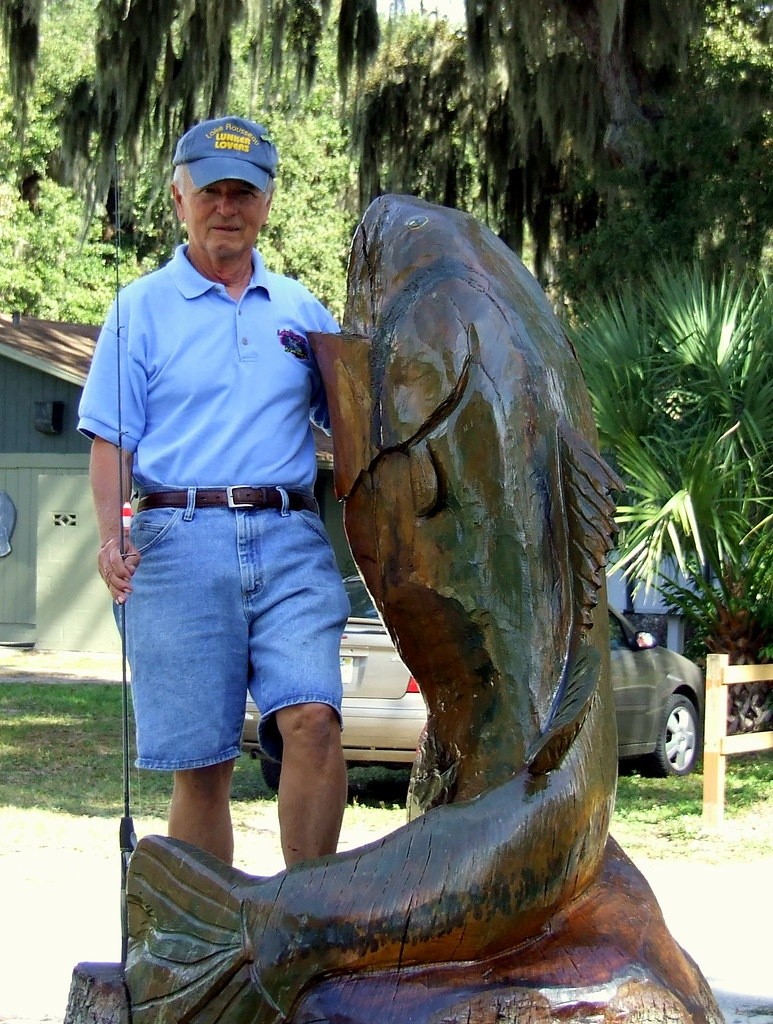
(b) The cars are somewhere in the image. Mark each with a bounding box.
[238,571,704,788]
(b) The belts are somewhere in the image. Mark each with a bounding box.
[137,486,319,513]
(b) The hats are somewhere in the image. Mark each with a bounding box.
[171,116,277,193]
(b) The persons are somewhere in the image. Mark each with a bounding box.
[76,119,351,869]
